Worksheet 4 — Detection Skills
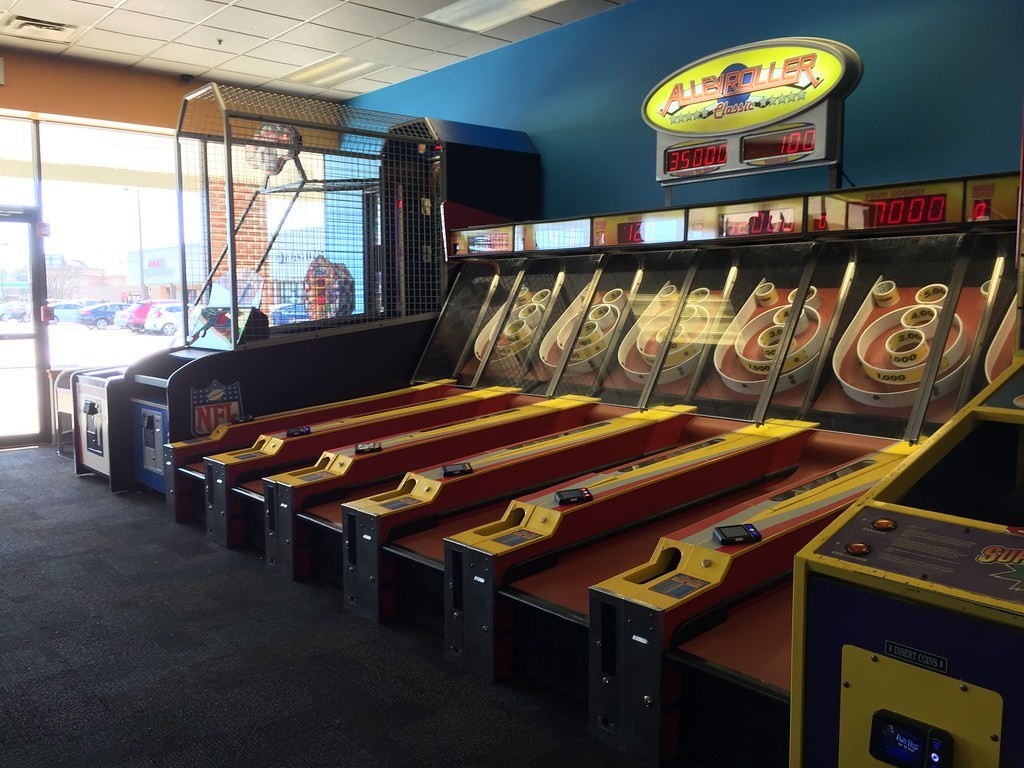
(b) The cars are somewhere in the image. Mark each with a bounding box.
[0,294,196,336]
